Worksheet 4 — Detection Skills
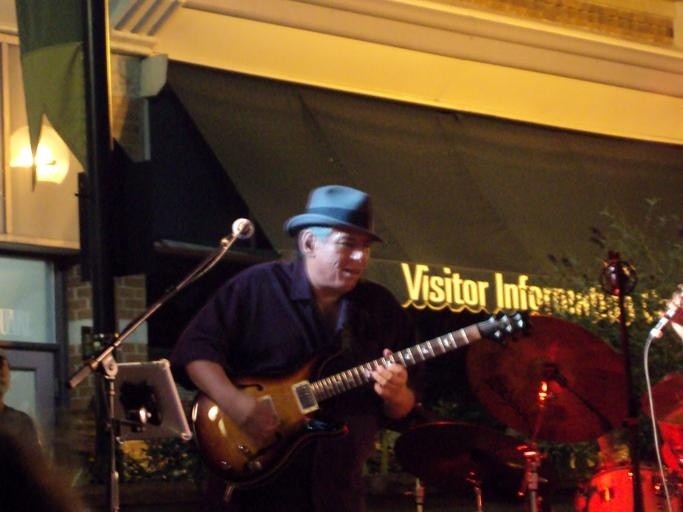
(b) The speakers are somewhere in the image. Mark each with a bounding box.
[78,160,153,276]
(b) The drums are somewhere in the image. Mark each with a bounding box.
[578,460,683,511]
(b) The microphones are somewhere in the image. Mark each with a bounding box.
[599,256,638,298]
[222,218,254,247]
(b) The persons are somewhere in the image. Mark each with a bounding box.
[172,184,432,512]
[0,348,40,456]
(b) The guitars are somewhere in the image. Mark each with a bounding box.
[186,307,535,487]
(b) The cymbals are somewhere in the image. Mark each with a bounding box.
[644,372,682,424]
[395,422,512,493]
[463,315,630,445]
[472,436,535,476]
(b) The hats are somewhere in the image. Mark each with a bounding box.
[284,185,390,248]
